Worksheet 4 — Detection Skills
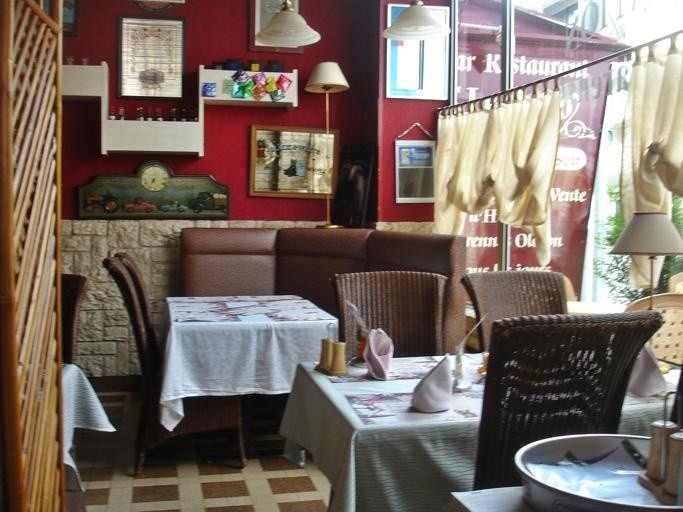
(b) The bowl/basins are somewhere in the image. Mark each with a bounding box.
[510,431,682,512]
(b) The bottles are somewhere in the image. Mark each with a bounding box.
[663,433,683,500]
[348,326,370,368]
[647,420,677,481]
[327,342,348,376]
[108,102,198,122]
[315,339,334,373]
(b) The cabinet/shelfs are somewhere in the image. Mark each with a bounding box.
[58,58,300,158]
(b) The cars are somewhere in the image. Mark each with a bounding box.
[79,191,227,216]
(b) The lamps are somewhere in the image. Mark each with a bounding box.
[382,0,451,41]
[256,0,321,47]
[609,210,683,348]
[305,61,350,224]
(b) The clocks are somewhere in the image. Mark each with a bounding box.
[140,163,171,192]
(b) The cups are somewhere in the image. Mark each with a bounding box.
[212,57,286,73]
[66,55,76,64]
[82,59,88,64]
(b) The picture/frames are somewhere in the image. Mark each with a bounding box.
[38,0,78,37]
[247,0,305,55]
[117,14,186,100]
[385,3,450,101]
[248,124,340,200]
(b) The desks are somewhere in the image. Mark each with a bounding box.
[443,481,532,512]
